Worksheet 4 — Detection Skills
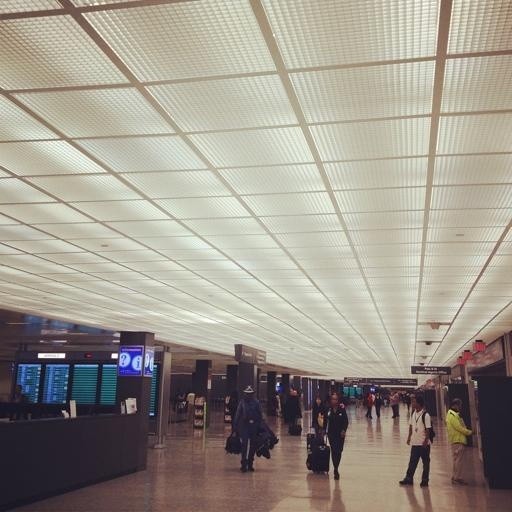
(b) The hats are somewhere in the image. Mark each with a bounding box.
[242,386,256,393]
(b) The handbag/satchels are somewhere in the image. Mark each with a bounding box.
[224,432,242,456]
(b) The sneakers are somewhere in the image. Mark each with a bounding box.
[452,478,469,485]
[334,470,339,480]
[399,478,429,486]
[240,466,254,472]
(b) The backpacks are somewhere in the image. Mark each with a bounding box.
[411,409,435,443]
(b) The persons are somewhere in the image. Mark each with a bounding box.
[330,480,346,512]
[367,418,400,443]
[309,389,351,480]
[12,384,24,404]
[355,389,411,418]
[399,394,475,487]
[269,386,305,434]
[404,485,433,512]
[231,385,265,473]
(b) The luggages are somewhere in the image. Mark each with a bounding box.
[288,417,303,435]
[306,433,330,473]
